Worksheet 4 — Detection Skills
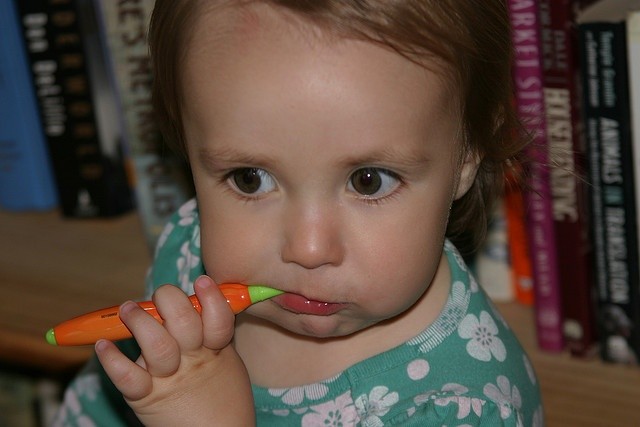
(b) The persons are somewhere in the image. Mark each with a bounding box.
[56,0,544,427]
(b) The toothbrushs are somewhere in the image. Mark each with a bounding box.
[45,282,286,347]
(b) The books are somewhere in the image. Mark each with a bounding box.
[476,0,638,363]
[1,0,185,250]
